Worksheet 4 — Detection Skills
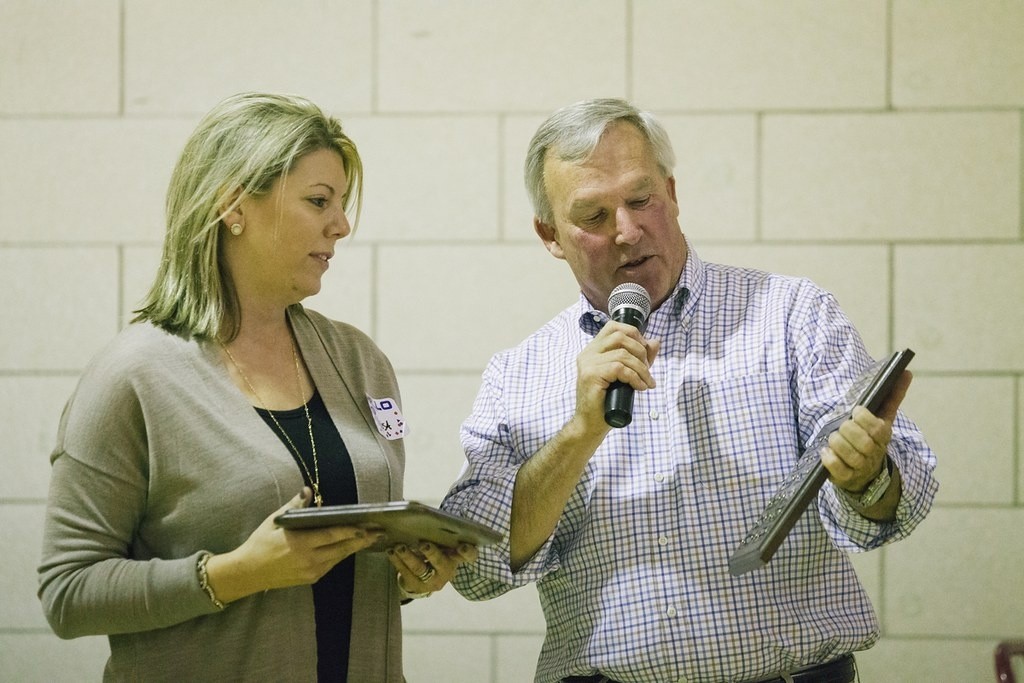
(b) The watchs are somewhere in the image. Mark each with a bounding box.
[842,453,891,511]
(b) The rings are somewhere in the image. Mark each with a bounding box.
[419,564,436,583]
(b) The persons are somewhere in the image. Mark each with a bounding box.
[33,89,480,683]
[436,96,938,683]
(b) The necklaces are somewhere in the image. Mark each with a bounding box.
[212,326,325,507]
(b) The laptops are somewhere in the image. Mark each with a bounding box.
[276,501,504,556]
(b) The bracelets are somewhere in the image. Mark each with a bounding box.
[396,571,433,599]
[195,552,229,613]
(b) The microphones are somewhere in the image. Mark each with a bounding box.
[603,281,651,429]
